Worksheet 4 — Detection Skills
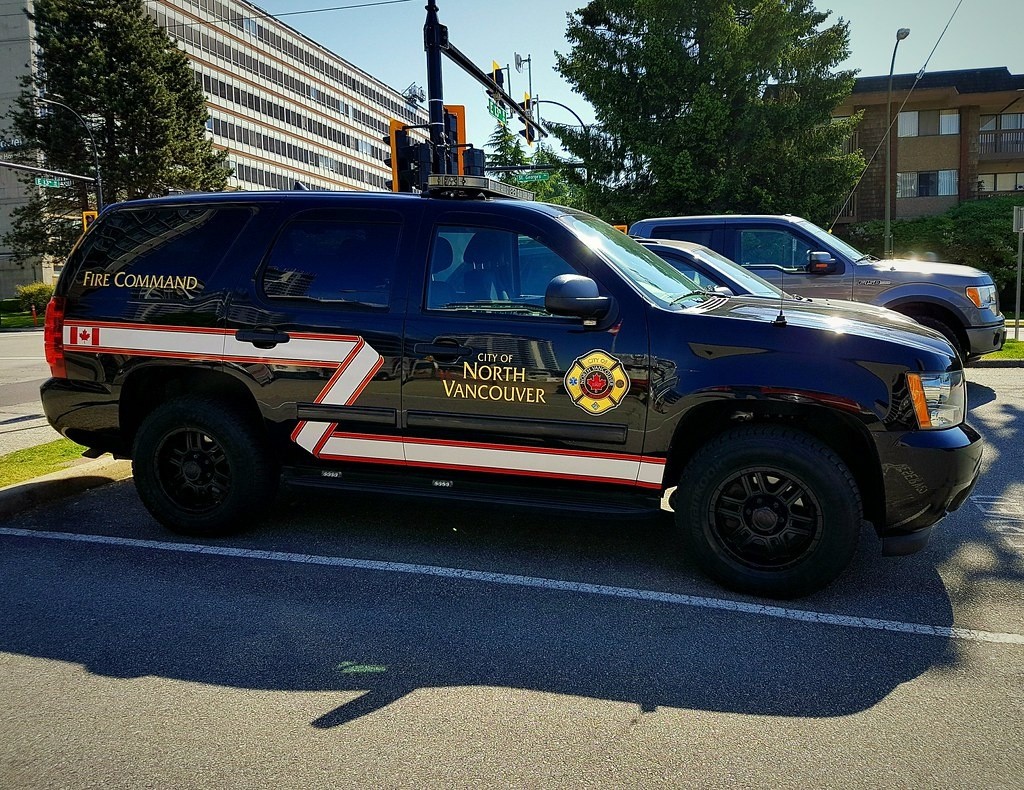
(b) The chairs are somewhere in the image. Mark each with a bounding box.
[462,230,509,301]
[429,237,459,306]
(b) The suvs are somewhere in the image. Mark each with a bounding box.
[41,173,989,602]
[510,212,1006,366]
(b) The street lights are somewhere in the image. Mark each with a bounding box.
[884,26,910,260]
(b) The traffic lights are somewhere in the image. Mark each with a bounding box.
[515,91,535,147]
[82,211,98,233]
[383,118,414,194]
[485,61,504,107]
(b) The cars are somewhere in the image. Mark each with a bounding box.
[458,240,919,334]
